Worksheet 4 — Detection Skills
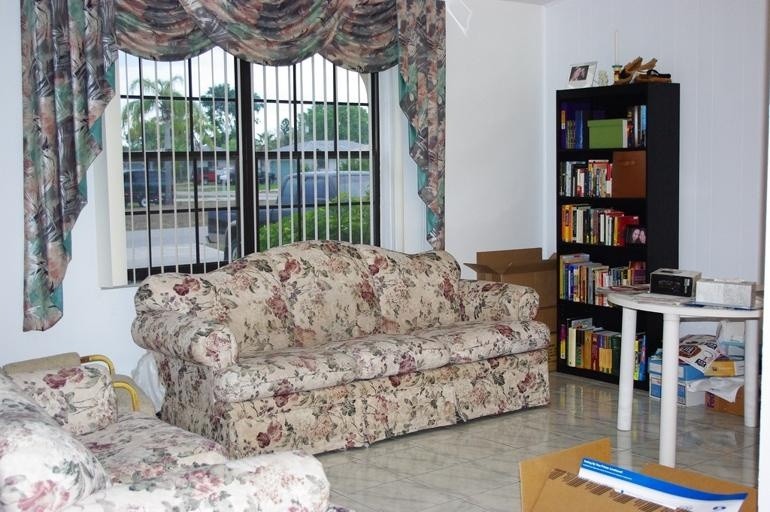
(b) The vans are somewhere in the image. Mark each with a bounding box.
[124,170,165,207]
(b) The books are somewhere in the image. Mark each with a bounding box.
[560,160,613,198]
[559,253,645,308]
[562,204,639,247]
[561,110,589,149]
[560,317,646,381]
[627,105,647,148]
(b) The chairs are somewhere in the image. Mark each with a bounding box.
[9,356,154,423]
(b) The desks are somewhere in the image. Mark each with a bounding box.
[607,281,763,467]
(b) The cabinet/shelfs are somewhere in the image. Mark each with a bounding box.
[556,82,680,393]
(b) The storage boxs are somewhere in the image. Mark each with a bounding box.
[463,248,557,372]
[519,436,757,511]
[648,334,760,420]
[650,268,757,309]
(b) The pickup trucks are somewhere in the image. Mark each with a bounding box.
[206,171,370,260]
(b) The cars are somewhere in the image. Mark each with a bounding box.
[190,167,275,185]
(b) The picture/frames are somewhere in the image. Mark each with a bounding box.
[567,61,597,87]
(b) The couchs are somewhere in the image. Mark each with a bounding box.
[0,354,331,512]
[131,239,551,456]
